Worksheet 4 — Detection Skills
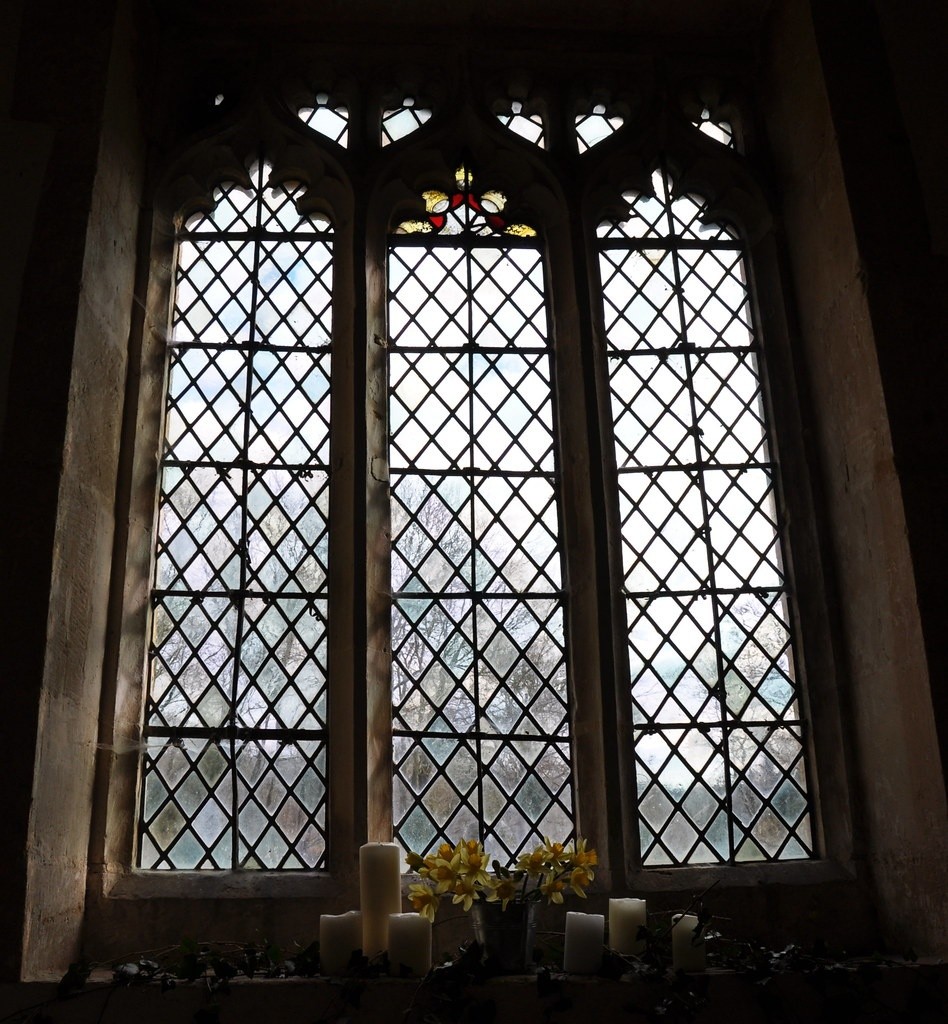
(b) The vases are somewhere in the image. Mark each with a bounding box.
[471,897,537,977]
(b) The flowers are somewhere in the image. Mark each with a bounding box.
[407,836,598,924]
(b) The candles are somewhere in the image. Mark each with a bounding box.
[608,898,646,955]
[564,911,605,973]
[320,910,360,972]
[387,911,432,975]
[360,840,401,960]
[673,914,699,973]
